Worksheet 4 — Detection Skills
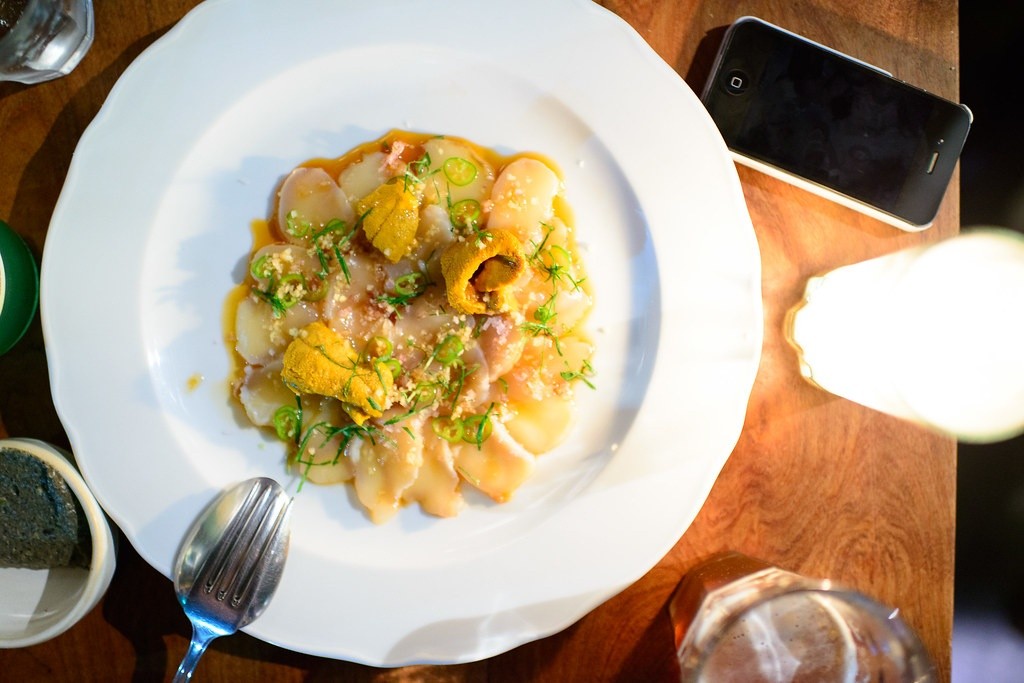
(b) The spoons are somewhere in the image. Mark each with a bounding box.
[169,477,294,683]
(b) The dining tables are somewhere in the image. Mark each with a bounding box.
[0,0,962,683]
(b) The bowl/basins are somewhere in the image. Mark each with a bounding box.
[0,438,118,649]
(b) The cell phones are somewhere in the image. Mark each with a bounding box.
[700,15,974,233]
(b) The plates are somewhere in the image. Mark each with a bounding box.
[40,0,764,668]
[0,219,39,358]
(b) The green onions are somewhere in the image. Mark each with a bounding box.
[251,158,495,442]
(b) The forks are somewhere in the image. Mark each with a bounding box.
[171,481,295,683]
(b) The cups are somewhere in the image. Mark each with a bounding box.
[0,0,94,84]
[668,552,937,683]
[781,226,1024,444]
